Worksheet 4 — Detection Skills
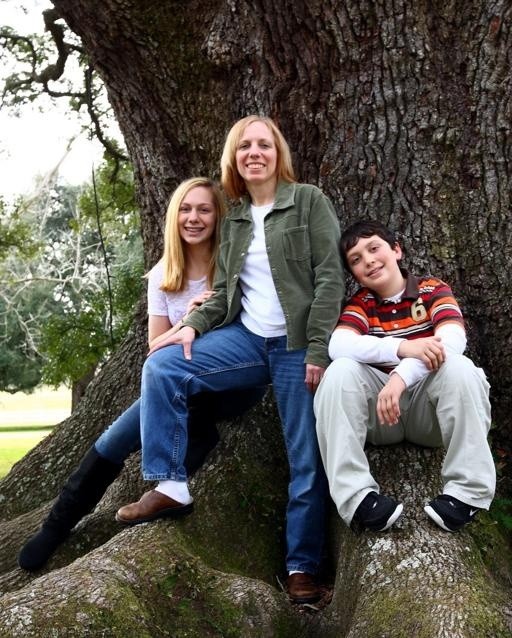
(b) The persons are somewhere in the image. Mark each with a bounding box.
[115,113,347,603]
[313,219,497,537]
[17,175,226,572]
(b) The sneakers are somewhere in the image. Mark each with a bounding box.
[354,491,403,532]
[423,494,482,532]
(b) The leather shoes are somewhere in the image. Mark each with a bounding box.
[114,490,195,525]
[287,570,320,604]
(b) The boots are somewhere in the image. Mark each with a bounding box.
[18,443,120,572]
